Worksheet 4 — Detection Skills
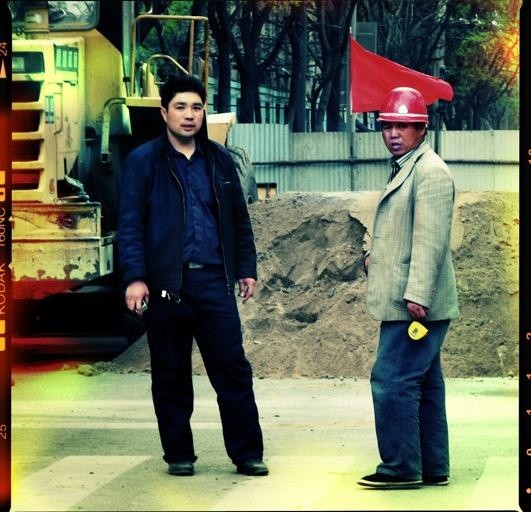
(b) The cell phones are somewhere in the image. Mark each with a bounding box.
[133,300,148,311]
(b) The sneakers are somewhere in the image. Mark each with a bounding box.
[422,474,449,486]
[356,472,425,488]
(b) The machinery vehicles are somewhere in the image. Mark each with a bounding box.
[11,1,259,352]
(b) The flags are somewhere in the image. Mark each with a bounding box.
[350,35,453,116]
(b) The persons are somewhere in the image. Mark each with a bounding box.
[359,84,454,486]
[119,76,268,479]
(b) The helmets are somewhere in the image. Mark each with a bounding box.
[376,86,430,126]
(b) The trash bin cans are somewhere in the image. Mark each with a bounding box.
[257,183,277,200]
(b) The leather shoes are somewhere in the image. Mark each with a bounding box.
[236,457,269,476]
[186,260,206,270]
[167,460,195,476]
